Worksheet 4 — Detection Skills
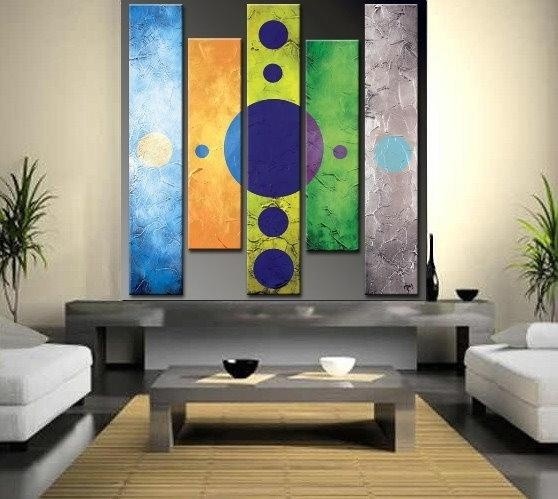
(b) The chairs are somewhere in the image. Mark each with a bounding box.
[0,316,94,451]
[464,321,558,444]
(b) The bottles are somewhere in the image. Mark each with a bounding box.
[427,234,439,302]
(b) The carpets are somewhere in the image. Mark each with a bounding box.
[41,392,526,499]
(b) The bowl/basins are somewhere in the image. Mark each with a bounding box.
[456,289,478,302]
[222,358,259,380]
[319,356,356,376]
